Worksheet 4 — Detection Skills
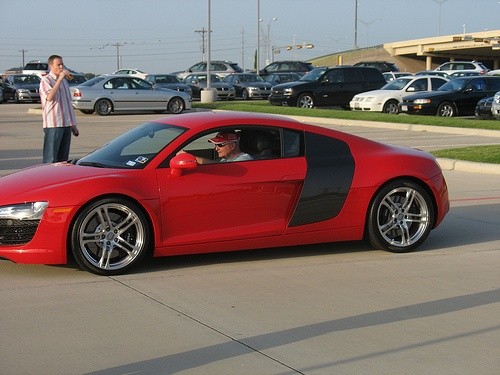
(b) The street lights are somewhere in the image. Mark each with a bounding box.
[258,17,278,65]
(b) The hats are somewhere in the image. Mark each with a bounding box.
[208,130,240,145]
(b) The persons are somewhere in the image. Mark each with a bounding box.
[38,54,80,163]
[176,129,254,164]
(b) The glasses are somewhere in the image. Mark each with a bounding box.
[215,141,237,147]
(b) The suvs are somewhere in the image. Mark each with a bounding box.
[267,66,387,109]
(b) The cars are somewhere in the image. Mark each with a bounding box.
[0,74,43,104]
[176,61,244,79]
[400,74,500,120]
[185,73,237,101]
[354,61,500,80]
[67,69,193,98]
[348,74,458,114]
[252,60,315,87]
[0,110,449,277]
[68,74,193,116]
[222,73,273,100]
